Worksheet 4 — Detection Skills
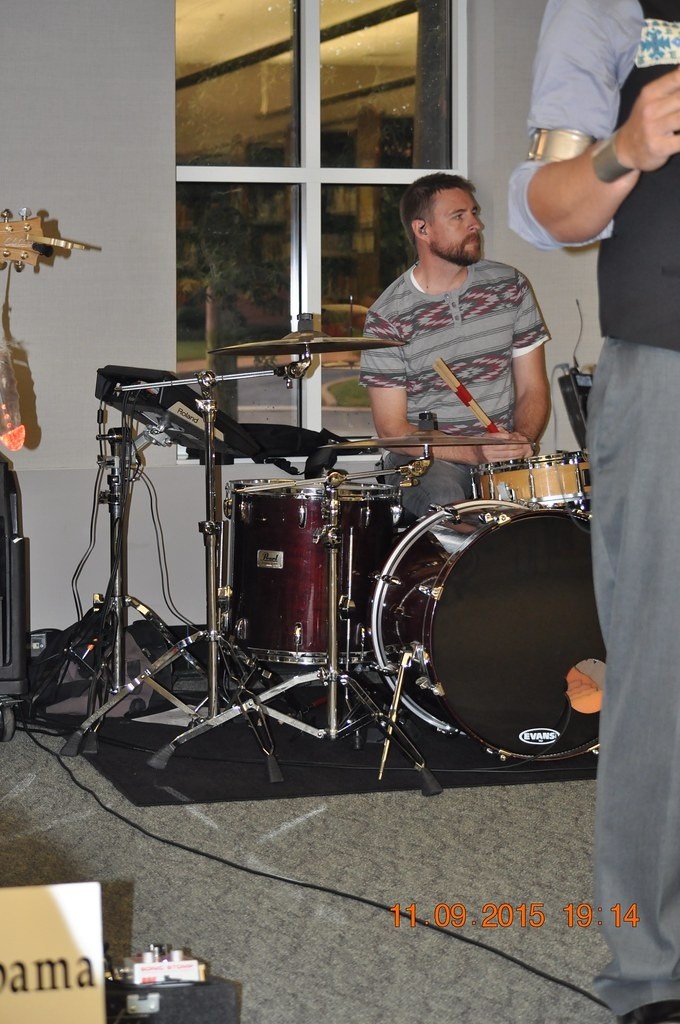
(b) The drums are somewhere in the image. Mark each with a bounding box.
[470,451,591,509]
[219,477,403,671]
[369,498,609,762]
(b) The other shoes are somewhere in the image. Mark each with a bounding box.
[618,999,680,1024]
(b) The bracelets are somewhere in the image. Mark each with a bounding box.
[592,133,635,184]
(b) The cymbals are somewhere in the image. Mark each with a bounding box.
[317,431,532,450]
[207,330,407,356]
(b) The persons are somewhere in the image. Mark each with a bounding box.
[359,173,553,521]
[508,1,680,1023]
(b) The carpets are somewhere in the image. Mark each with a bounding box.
[39,626,595,809]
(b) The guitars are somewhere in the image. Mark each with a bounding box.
[1,205,91,273]
